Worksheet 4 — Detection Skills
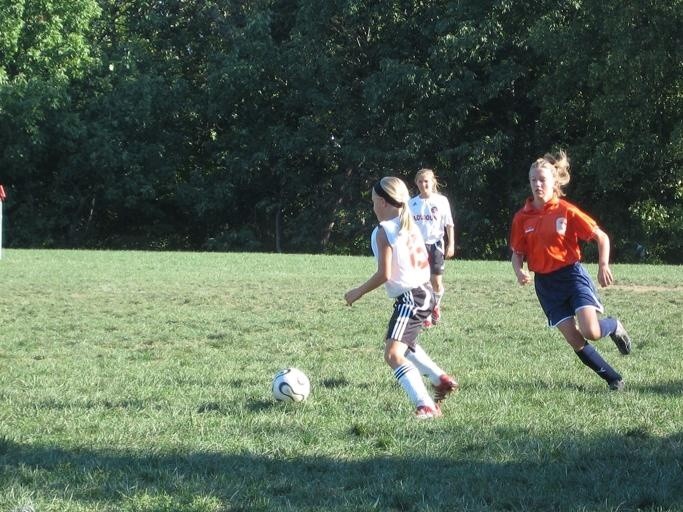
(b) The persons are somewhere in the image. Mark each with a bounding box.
[343,174,459,421]
[509,148,633,394]
[407,168,454,330]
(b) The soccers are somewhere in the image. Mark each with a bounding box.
[273,368,309,402]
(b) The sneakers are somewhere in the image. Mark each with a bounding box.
[607,316,631,355]
[431,374,459,405]
[413,402,442,420]
[608,380,625,395]
[431,306,441,325]
[422,319,431,328]
